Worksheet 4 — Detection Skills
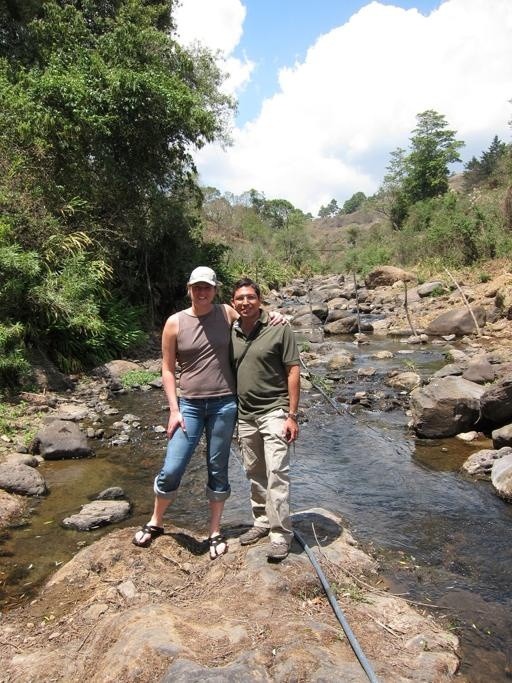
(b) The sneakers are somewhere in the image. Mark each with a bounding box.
[240,527,270,545]
[265,543,290,559]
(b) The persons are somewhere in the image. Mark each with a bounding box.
[229,275,303,561]
[130,264,290,559]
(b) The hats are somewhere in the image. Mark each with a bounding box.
[187,266,217,287]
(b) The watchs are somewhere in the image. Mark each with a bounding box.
[287,411,299,420]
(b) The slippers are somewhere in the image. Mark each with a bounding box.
[132,523,164,547]
[208,534,227,560]
